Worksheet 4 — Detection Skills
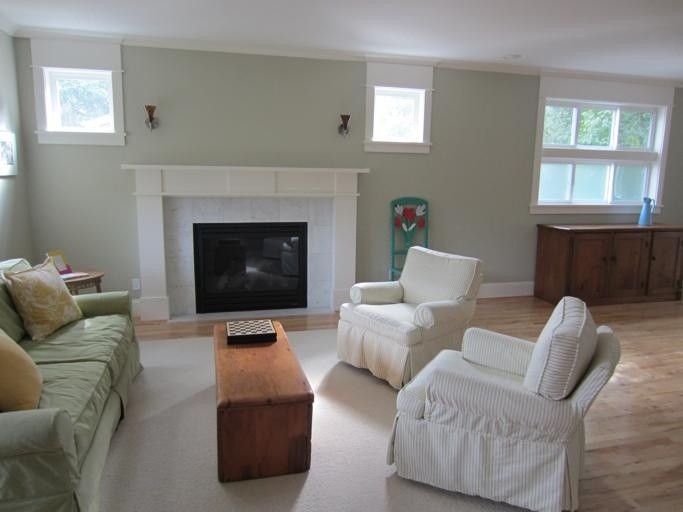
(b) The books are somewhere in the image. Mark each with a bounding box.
[46,251,71,274]
[61,272,91,281]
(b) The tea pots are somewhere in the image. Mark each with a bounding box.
[638,196,655,227]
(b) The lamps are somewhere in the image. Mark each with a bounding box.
[335,112,352,137]
[143,104,157,133]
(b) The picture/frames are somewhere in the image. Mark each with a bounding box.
[0,131,18,177]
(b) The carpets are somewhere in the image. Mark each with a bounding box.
[96,327,540,512]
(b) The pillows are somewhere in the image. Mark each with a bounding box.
[0,327,44,414]
[521,295,599,403]
[0,256,86,346]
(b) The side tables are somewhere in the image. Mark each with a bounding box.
[61,270,105,295]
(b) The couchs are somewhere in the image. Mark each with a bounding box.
[0,256,145,512]
[380,323,622,512]
[334,243,487,392]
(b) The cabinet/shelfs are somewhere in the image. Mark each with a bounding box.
[533,222,653,308]
[644,225,682,304]
